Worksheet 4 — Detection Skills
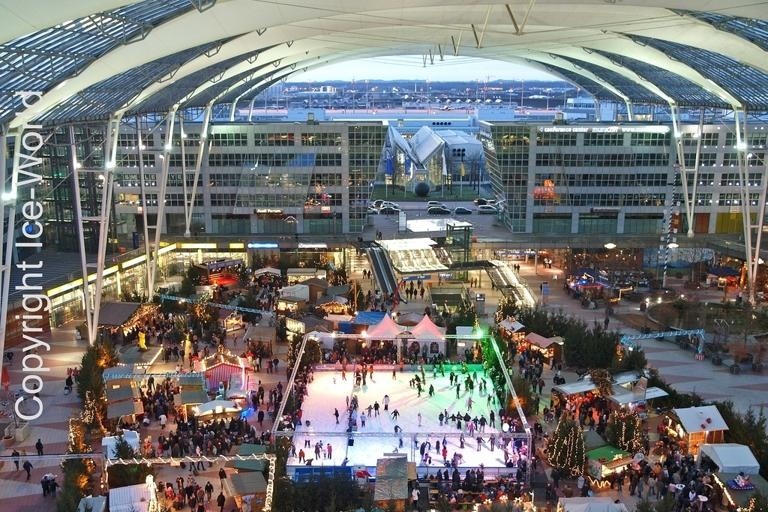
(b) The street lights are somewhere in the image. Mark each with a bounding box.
[602,241,617,330]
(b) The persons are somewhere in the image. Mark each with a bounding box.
[378,232,382,239]
[61,255,729,512]
[40,475,50,496]
[33,383,39,397]
[735,471,749,485]
[22,459,33,480]
[12,449,20,471]
[48,477,60,498]
[35,438,44,455]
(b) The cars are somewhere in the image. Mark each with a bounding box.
[366,199,401,215]
[426,197,499,216]
